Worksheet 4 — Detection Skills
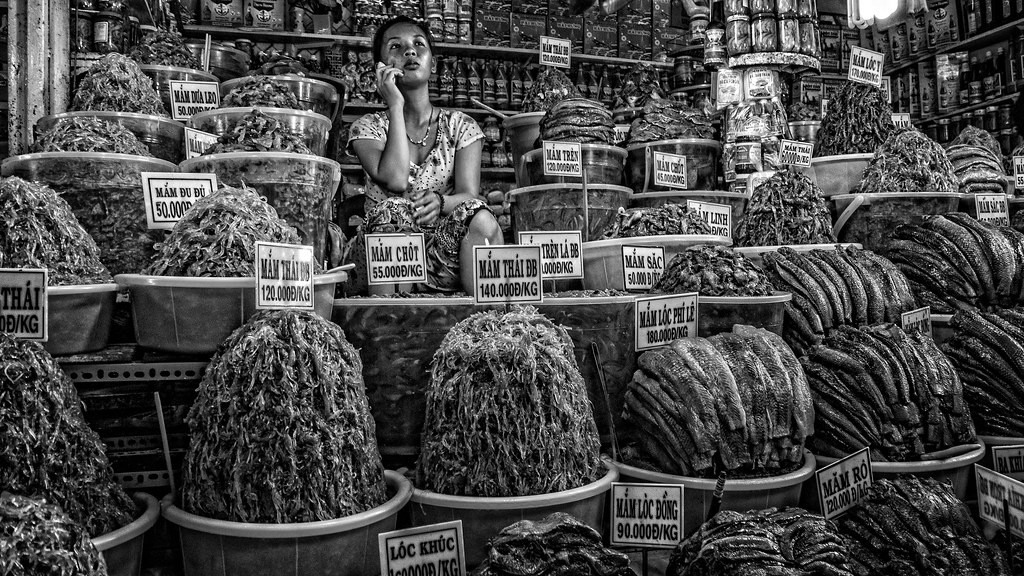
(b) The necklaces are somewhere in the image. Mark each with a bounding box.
[406,106,434,146]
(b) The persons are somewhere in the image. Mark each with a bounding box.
[340,16,505,296]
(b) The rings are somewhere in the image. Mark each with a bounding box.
[375,79,377,81]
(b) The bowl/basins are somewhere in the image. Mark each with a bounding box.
[0,65,347,351]
[506,111,969,336]
[89,434,1024,576]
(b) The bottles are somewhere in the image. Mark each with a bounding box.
[426,56,535,108]
[575,60,623,108]
[960,0,1024,106]
[482,117,509,168]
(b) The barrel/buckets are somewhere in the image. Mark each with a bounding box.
[334,289,654,444]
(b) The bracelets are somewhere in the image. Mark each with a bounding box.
[433,191,445,217]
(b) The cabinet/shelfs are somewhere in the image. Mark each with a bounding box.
[70,0,1024,185]
